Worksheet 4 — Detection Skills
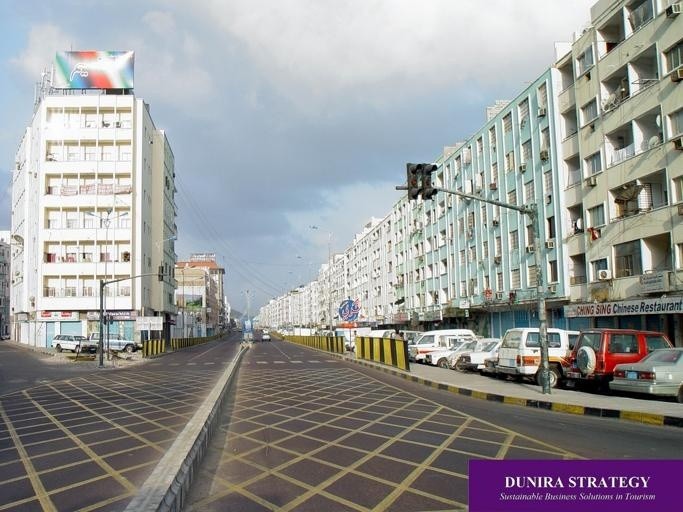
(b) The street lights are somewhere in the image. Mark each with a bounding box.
[181,265,196,340]
[308,224,333,336]
[289,256,324,336]
[84,208,128,353]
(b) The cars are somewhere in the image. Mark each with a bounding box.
[426,337,502,373]
[1,334,10,339]
[261,329,272,342]
[609,347,683,403]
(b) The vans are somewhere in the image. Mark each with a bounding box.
[51,334,100,353]
[89,332,138,352]
[494,327,599,388]
[368,329,477,363]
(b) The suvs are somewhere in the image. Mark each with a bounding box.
[566,327,674,390]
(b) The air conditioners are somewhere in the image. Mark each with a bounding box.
[495,3,683,300]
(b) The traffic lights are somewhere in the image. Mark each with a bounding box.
[158,266,164,281]
[407,163,438,200]
[104,314,113,325]
